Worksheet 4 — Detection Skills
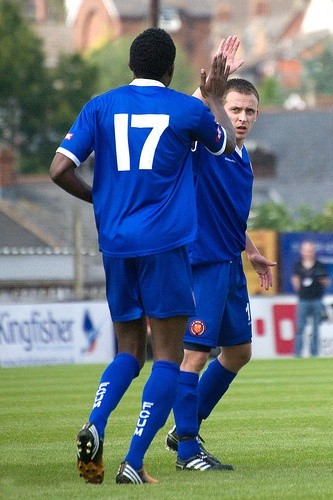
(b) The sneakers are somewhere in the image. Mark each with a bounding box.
[115,461,159,485]
[174,452,217,472]
[165,425,221,470]
[76,423,104,484]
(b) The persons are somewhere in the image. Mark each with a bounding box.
[47,28,237,485]
[290,239,330,358]
[165,34,277,471]
